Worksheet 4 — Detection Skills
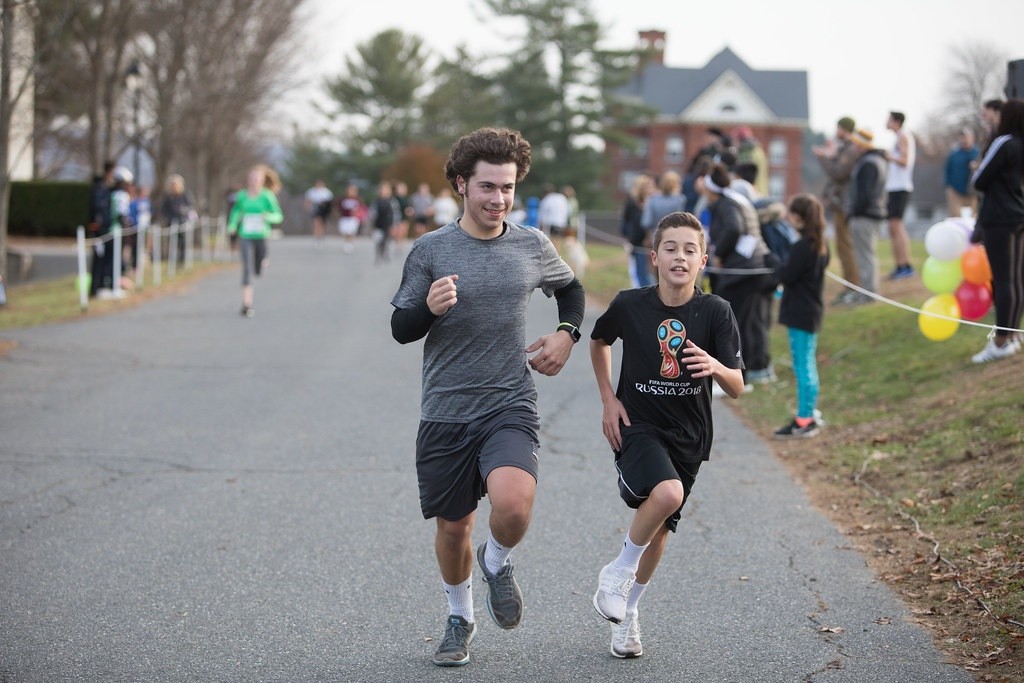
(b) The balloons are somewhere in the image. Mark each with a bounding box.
[918,219,992,340]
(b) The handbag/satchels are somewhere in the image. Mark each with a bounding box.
[719,239,763,284]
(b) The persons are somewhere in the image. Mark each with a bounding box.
[304,179,333,239]
[89,161,157,298]
[163,173,195,264]
[589,212,746,658]
[391,127,585,664]
[506,184,578,249]
[971,101,1024,363]
[623,126,792,395]
[812,112,914,305]
[371,182,461,261]
[226,165,284,316]
[337,183,368,244]
[943,127,979,217]
[764,197,830,438]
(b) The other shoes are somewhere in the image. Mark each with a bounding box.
[971,336,1020,364]
[774,419,820,439]
[748,363,775,387]
[891,265,915,280]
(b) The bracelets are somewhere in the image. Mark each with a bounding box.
[557,325,581,343]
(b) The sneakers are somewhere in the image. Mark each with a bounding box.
[593,561,636,624]
[610,610,642,658]
[433,615,476,665]
[477,542,523,629]
